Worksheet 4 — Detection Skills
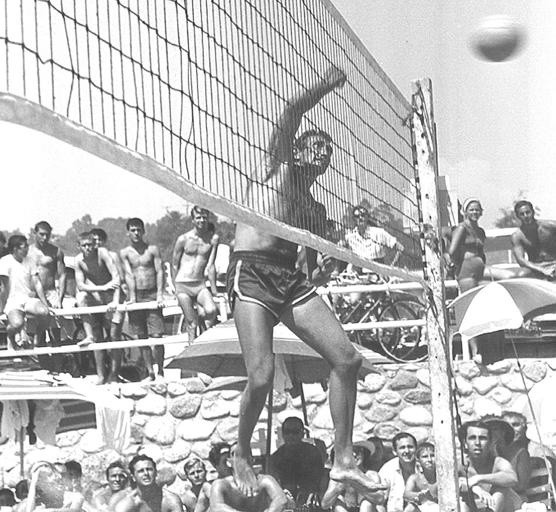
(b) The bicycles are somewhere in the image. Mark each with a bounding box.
[318,271,434,360]
[23,314,147,383]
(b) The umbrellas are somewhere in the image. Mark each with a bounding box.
[163,316,382,474]
[446,268,556,340]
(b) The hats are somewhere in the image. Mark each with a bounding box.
[350,435,374,456]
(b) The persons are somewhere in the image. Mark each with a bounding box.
[0,205,235,386]
[229,66,390,497]
[511,199,556,281]
[0,411,556,512]
[317,206,403,303]
[449,198,485,365]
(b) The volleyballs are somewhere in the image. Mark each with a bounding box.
[469,16,519,61]
